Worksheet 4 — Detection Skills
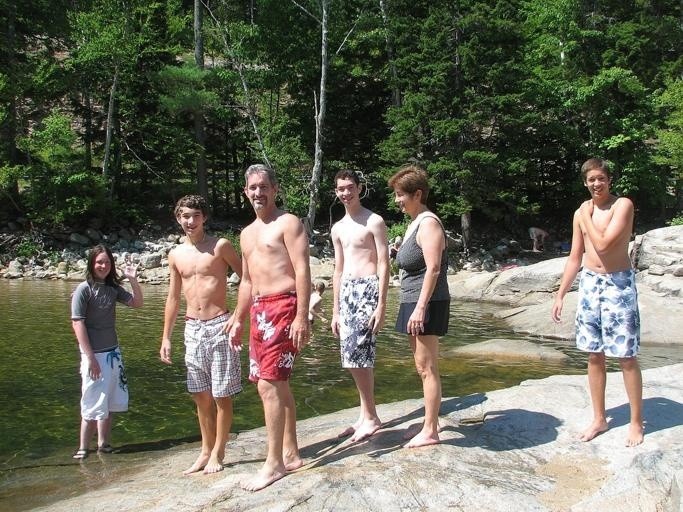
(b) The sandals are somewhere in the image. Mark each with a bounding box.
[97,444,120,453]
[73,449,89,459]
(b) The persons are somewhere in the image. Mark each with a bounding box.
[158,194,244,476]
[551,155,646,448]
[387,237,402,258]
[329,168,391,444]
[308,282,327,328]
[226,162,311,492]
[528,226,549,253]
[388,166,451,448]
[70,243,143,459]
